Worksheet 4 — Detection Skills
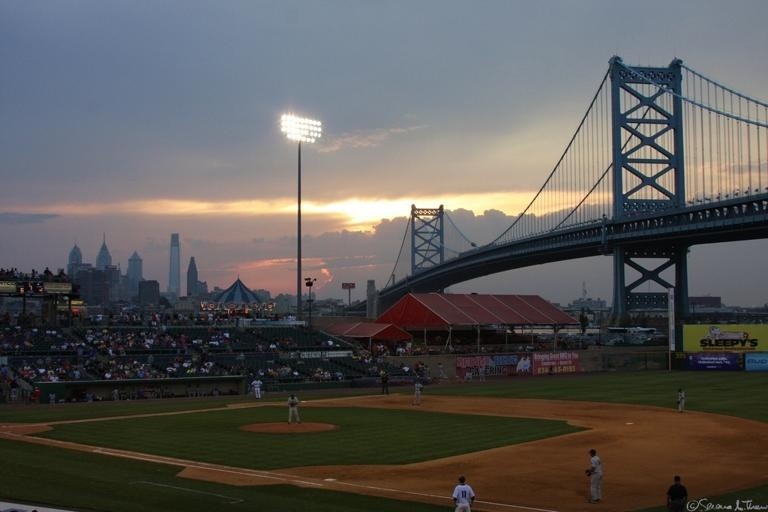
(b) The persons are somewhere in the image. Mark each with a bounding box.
[674,387,686,412]
[1,306,590,406]
[583,449,604,505]
[286,394,302,424]
[451,476,474,512]
[664,476,688,511]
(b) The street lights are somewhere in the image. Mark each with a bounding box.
[280,114,322,322]
[305,278,316,322]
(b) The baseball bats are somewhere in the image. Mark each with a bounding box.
[298,400,311,403]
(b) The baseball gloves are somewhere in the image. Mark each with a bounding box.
[585,468,593,477]
[420,389,423,392]
